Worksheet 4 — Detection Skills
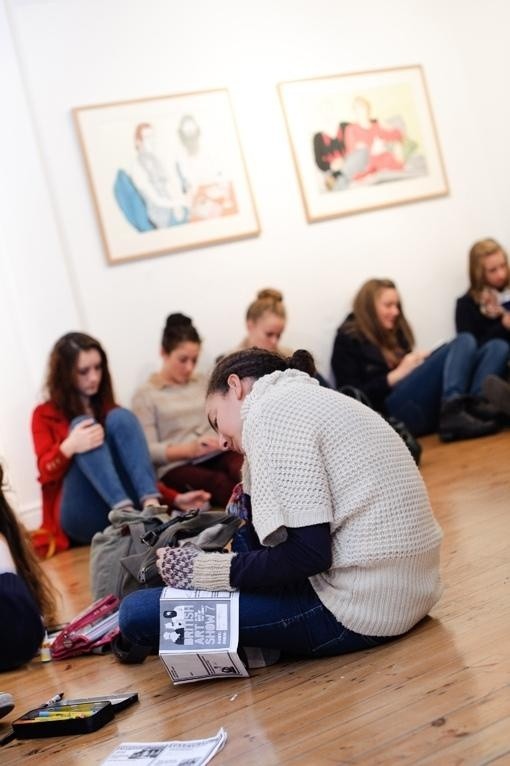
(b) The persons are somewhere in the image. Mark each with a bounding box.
[234,287,334,388]
[454,236,509,346]
[0,460,61,725]
[178,111,235,220]
[26,331,212,568]
[129,310,244,511]
[111,345,446,670]
[328,276,510,443]
[110,121,191,230]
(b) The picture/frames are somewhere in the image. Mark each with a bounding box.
[275,62,452,225]
[72,62,263,268]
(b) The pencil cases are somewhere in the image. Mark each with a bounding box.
[12,692,138,738]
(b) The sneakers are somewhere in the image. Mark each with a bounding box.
[481,374,510,414]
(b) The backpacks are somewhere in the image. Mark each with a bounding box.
[90,503,183,602]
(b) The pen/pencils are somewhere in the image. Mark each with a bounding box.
[16,693,92,723]
[193,430,209,446]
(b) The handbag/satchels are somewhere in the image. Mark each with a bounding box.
[49,594,122,660]
[136,508,242,584]
[340,385,422,464]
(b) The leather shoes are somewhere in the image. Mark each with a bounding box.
[467,397,503,419]
[441,410,500,442]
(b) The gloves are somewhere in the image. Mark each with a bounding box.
[155,545,239,593]
